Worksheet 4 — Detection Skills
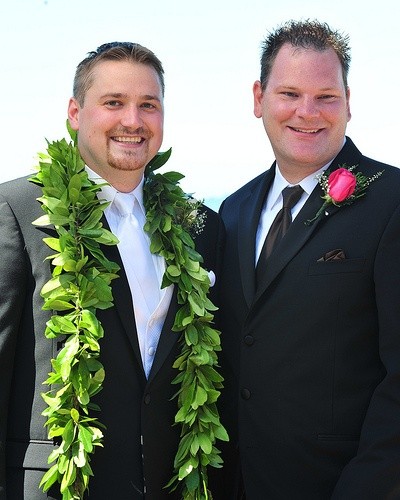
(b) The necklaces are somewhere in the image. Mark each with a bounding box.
[26,116,229,498]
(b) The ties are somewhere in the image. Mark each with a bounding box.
[114,191,165,314]
[255,186,310,282]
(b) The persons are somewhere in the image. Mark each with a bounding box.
[219,18,400,500]
[0,42,230,499]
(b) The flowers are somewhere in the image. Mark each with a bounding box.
[177,192,208,239]
[303,163,386,227]
